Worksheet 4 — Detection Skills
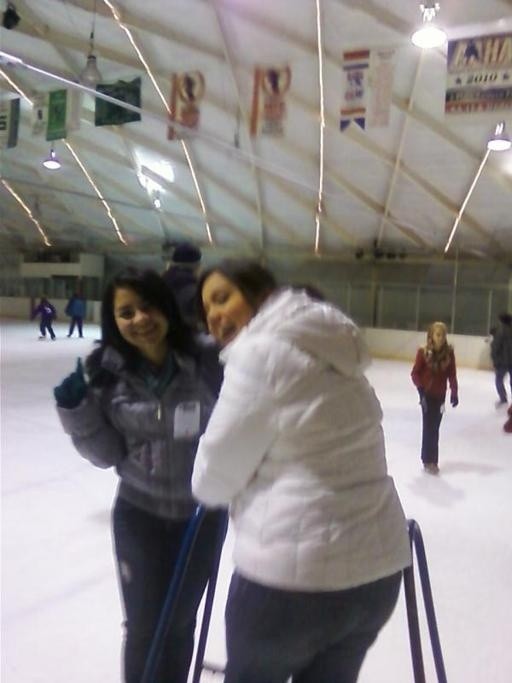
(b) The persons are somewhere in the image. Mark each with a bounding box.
[189,257,414,683]
[410,320,458,473]
[30,295,57,340]
[490,310,511,406]
[63,288,86,337]
[55,263,326,683]
[159,240,209,326]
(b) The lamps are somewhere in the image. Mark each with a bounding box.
[485,120,512,151]
[42,140,63,171]
[409,0,452,48]
[80,0,106,84]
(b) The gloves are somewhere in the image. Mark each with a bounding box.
[53,357,87,409]
